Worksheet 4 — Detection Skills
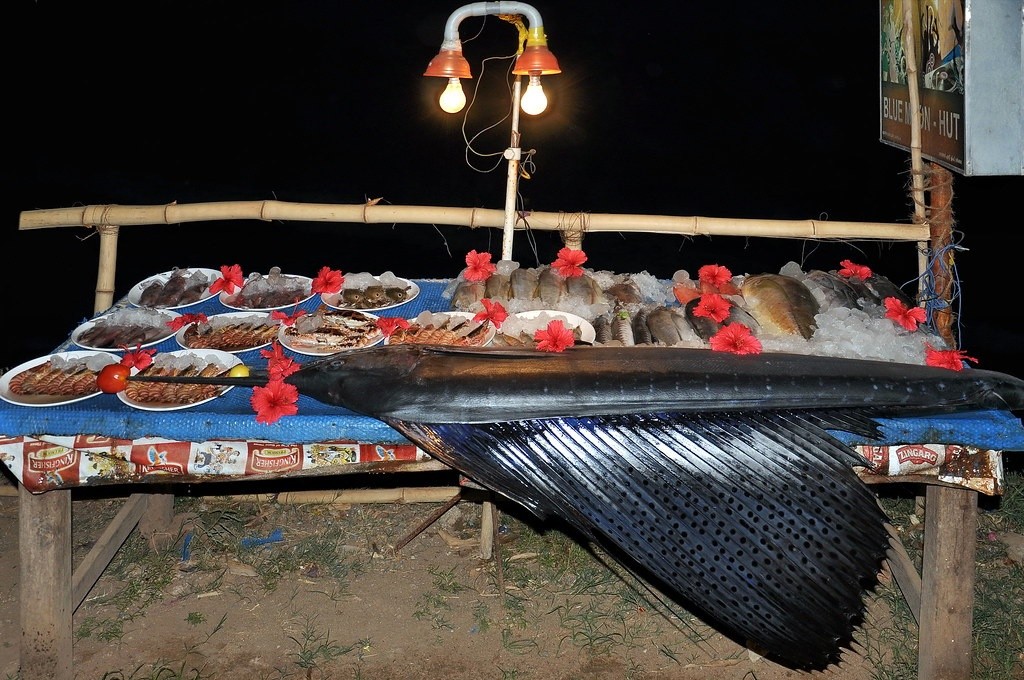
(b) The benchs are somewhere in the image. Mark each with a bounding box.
[1,201,1024,680]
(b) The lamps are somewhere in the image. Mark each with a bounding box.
[422,1,561,115]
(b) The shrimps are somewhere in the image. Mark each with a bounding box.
[8,360,99,396]
[388,317,584,347]
[124,361,244,405]
[183,321,281,349]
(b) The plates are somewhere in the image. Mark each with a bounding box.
[384,312,496,350]
[72,310,185,352]
[277,309,389,357]
[115,349,244,411]
[493,310,596,349]
[0,351,122,407]
[128,268,226,309]
[174,312,282,355]
[320,275,420,311]
[219,273,317,312]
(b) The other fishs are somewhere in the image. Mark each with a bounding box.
[75,318,164,349]
[445,266,928,348]
[138,274,414,308]
